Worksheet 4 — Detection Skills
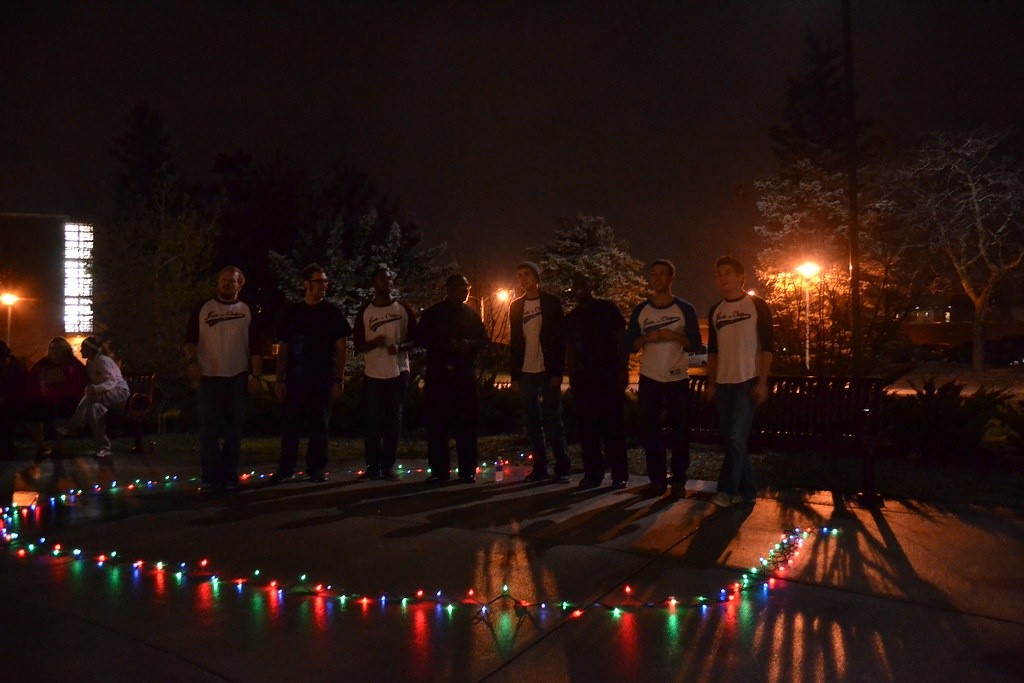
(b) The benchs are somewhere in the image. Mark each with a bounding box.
[55,368,159,455]
[644,374,885,502]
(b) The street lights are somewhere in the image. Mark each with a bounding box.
[1,292,16,346]
[794,257,822,370]
[469,290,509,323]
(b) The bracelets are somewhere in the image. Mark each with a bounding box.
[276,378,284,384]
[252,375,262,381]
[188,356,197,365]
[335,377,343,385]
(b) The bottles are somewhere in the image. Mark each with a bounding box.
[271,340,280,356]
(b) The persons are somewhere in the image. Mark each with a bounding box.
[270,265,352,483]
[0,340,32,506]
[354,263,418,481]
[56,336,130,457]
[704,255,773,507]
[20,336,86,463]
[509,261,572,483]
[183,265,263,489]
[565,270,629,488]
[413,274,491,483]
[629,260,702,498]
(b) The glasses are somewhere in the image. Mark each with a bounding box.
[311,279,329,285]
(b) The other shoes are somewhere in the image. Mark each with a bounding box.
[197,479,237,494]
[525,470,547,481]
[670,485,686,497]
[311,472,327,481]
[613,480,629,489]
[557,472,570,481]
[578,478,600,488]
[358,467,381,481]
[272,470,294,482]
[426,470,450,483]
[711,492,731,506]
[644,481,666,495]
[94,447,110,458]
[733,491,747,503]
[460,471,475,483]
[56,426,77,437]
[382,467,401,481]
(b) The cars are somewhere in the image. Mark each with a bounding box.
[409,342,513,390]
[935,339,1024,367]
[688,346,708,366]
